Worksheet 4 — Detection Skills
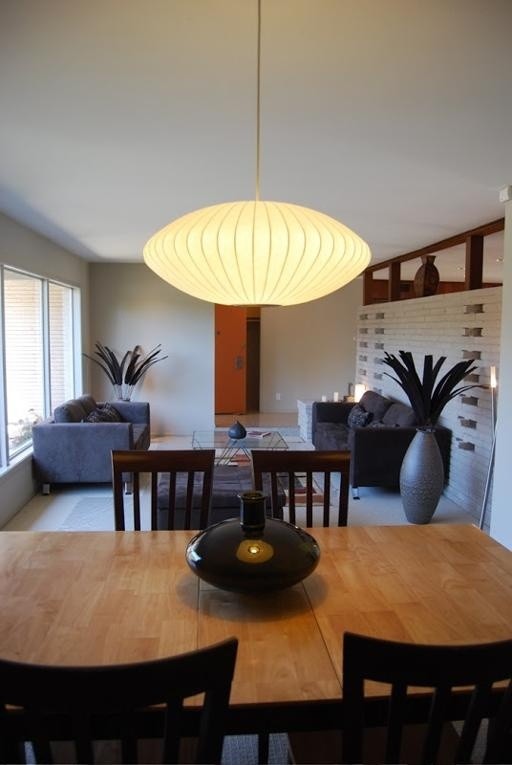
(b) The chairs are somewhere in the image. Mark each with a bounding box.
[0,635,238,765]
[287,627,511,765]
[109,447,216,532]
[251,448,352,528]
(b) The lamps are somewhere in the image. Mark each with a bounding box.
[478,365,499,527]
[353,383,366,403]
[142,0,372,309]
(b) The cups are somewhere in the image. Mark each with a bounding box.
[333,392,339,402]
[322,395,326,402]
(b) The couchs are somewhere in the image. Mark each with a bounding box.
[32,392,151,498]
[311,390,452,499]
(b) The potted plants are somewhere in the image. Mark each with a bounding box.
[379,348,481,528]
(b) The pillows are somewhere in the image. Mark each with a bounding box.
[348,404,367,430]
[365,419,400,428]
[100,402,129,422]
[84,407,111,423]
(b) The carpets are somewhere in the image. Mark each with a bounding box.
[214,435,333,509]
